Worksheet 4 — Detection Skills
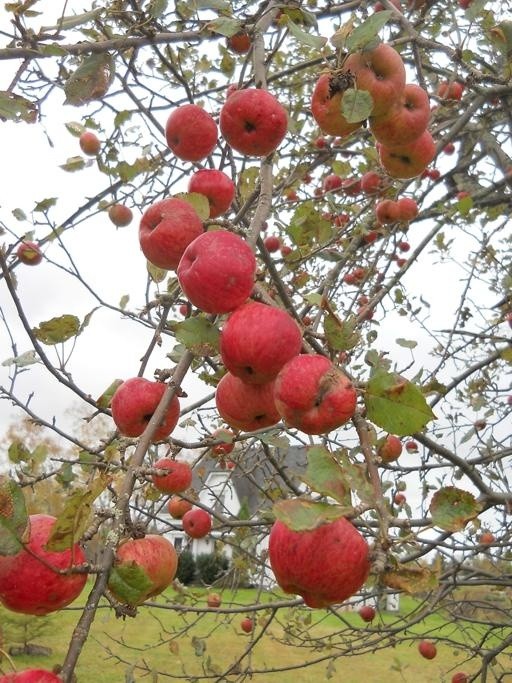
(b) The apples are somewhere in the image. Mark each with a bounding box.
[0,1,508,683]
